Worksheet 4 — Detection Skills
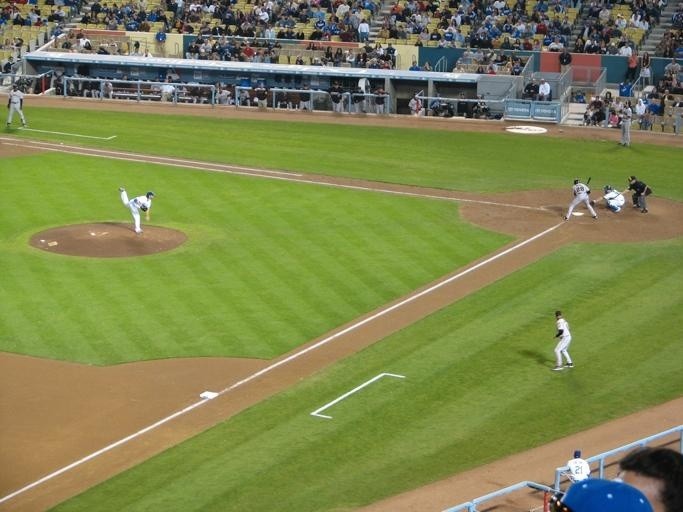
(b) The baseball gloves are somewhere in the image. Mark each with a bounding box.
[141,205,147,211]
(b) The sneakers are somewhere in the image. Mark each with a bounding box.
[551,363,574,371]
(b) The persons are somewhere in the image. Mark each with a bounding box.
[117,186,153,235]
[619,101,633,146]
[0,0,682,130]
[566,448,591,482]
[614,443,683,511]
[562,178,598,222]
[589,184,625,213]
[620,175,653,214]
[552,310,574,370]
[549,477,653,512]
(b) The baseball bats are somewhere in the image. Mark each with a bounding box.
[586,177,591,186]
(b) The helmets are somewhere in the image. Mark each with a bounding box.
[574,180,580,184]
[560,479,652,512]
[556,311,561,316]
[604,185,611,190]
[147,192,153,197]
[628,176,635,181]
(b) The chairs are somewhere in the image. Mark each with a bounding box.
[1,0,682,130]
